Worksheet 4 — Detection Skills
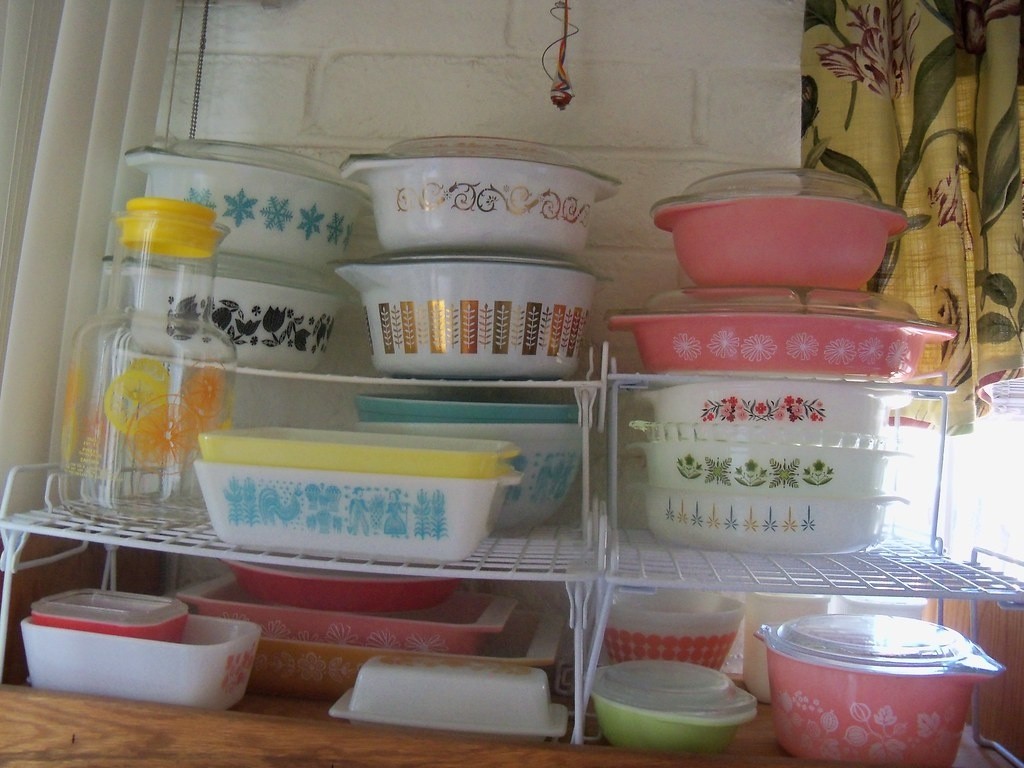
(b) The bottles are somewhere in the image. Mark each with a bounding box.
[58,196,237,526]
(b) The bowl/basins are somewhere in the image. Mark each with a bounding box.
[335,258,596,379]
[604,287,958,380]
[583,659,758,753]
[192,459,522,562]
[355,393,585,534]
[753,613,1007,768]
[626,382,931,556]
[339,136,623,258]
[98,250,341,373]
[603,590,745,671]
[197,427,522,478]
[649,169,909,292]
[124,139,373,275]
